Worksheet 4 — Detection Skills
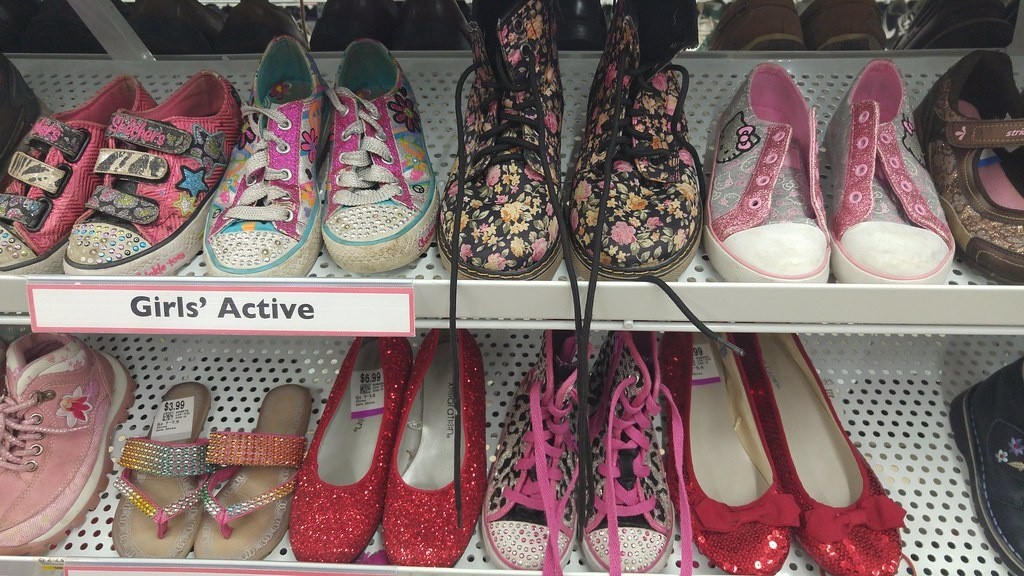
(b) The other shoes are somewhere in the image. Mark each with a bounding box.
[0,328,1024,576]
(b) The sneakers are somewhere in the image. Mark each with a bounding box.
[0,2,1024,286]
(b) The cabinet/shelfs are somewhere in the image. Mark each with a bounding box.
[0,52,1024,576]
